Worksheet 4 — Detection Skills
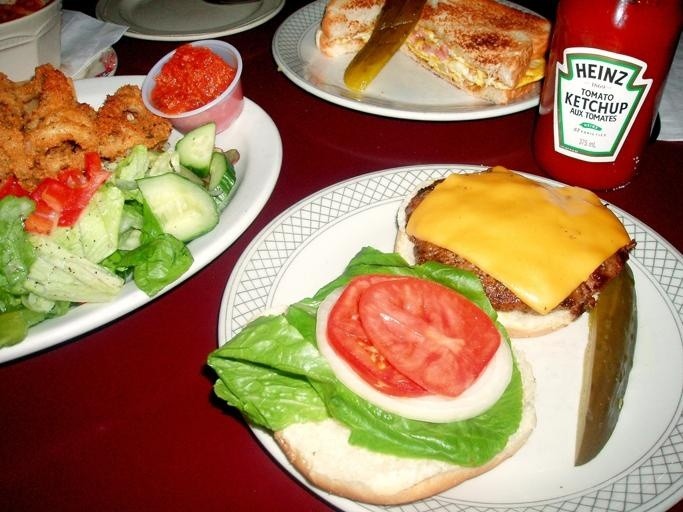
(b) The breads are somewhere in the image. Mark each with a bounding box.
[392,178,602,339]
[316,0,553,107]
[204,342,537,507]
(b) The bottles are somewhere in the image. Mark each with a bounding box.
[528,1,682,192]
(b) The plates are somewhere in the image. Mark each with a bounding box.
[216,166,682,512]
[75,44,118,78]
[1,73,281,361]
[272,1,555,123]
[95,1,283,44]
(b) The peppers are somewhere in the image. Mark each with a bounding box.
[574,249,638,464]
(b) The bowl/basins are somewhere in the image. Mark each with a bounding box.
[0,2,65,82]
[141,38,244,135]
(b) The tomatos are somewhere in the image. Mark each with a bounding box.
[325,274,503,401]
